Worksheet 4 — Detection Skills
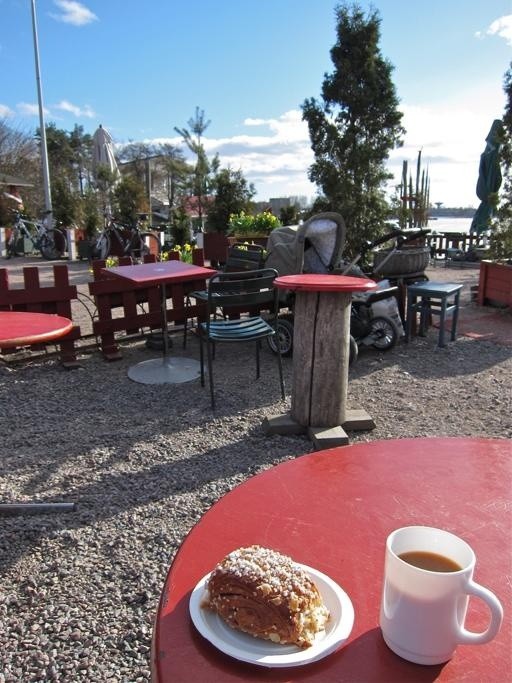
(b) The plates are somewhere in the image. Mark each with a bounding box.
[189,561,355,668]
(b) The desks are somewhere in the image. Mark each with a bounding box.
[263,274,379,450]
[150,437,511,681]
[99,260,218,384]
[0,310,75,512]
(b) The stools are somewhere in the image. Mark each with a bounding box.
[383,273,433,330]
[405,281,464,348]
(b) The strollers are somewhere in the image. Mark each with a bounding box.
[264,210,434,364]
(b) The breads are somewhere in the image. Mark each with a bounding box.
[199,543,332,649]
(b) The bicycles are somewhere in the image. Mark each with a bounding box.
[90,208,162,266]
[0,205,66,261]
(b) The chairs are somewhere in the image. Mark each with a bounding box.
[182,242,264,360]
[198,267,286,411]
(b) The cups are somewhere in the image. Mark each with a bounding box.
[379,525,503,667]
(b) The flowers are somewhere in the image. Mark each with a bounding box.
[226,209,284,237]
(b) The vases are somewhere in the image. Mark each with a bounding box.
[237,237,268,247]
